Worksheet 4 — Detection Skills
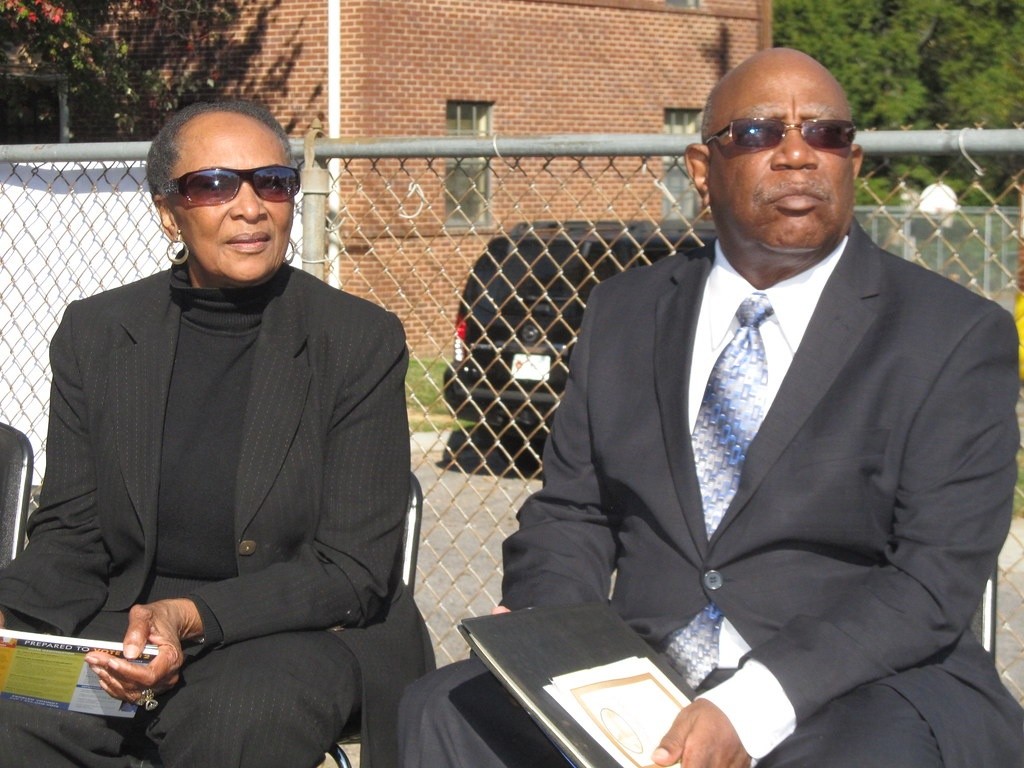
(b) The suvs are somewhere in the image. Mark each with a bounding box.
[443,220,716,429]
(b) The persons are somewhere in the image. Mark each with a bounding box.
[2,90,438,768]
[390,46,1018,768]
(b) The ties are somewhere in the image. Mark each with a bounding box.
[661,294,774,690]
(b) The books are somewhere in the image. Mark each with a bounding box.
[0,629,159,717]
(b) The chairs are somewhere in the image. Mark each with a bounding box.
[0,422,34,578]
[327,471,425,768]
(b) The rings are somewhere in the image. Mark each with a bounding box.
[134,687,159,710]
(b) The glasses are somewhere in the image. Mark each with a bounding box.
[705,117,856,149]
[158,165,301,206]
